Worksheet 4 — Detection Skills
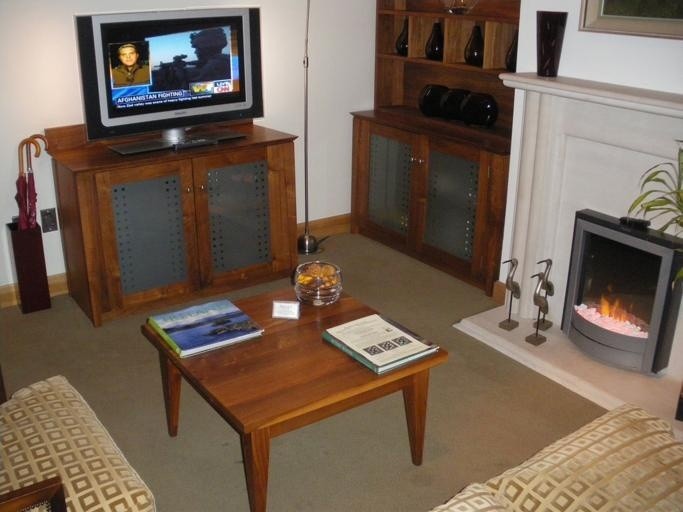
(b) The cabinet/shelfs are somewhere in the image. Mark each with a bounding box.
[350,111,510,297]
[45,126,299,328]
[374,1,519,155]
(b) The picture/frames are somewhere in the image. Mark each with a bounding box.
[578,0,682,40]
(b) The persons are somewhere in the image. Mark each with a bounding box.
[109,42,148,86]
[170,27,233,88]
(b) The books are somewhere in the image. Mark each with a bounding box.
[146,298,267,361]
[321,313,440,375]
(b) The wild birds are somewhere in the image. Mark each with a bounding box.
[536,257,555,322]
[530,272,549,339]
[501,257,521,324]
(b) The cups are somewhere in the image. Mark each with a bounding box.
[293,260,345,308]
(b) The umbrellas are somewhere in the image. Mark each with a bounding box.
[25,134,48,228]
[14,138,40,231]
